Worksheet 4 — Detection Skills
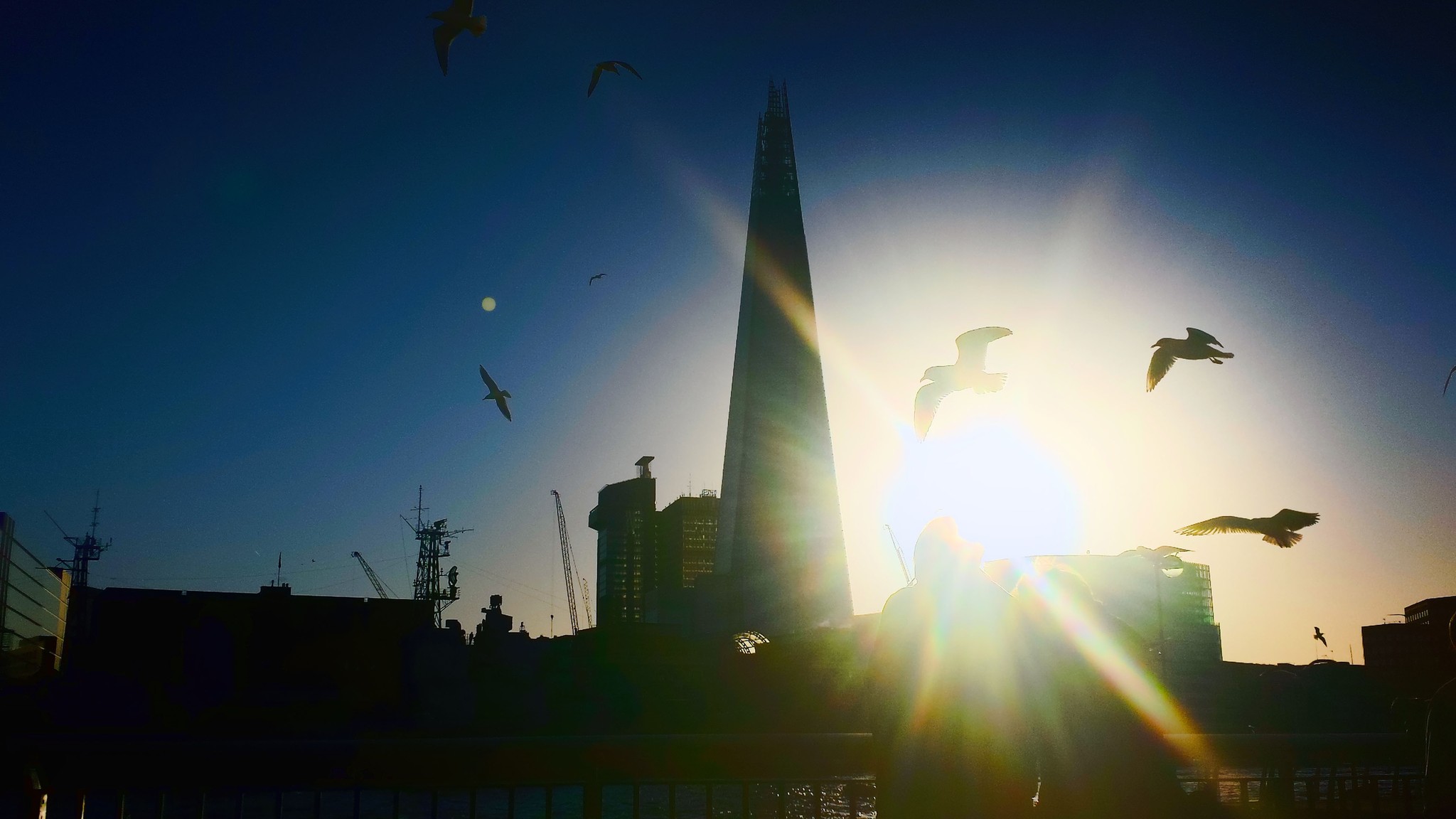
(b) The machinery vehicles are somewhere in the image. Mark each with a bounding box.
[350,549,398,600]
[549,489,595,633]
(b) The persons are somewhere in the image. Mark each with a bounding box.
[469,632,473,644]
[868,520,1180,819]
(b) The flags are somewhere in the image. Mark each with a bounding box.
[279,552,281,568]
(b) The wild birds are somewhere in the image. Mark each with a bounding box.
[1144,324,1235,396]
[586,57,642,98]
[1313,626,1328,647]
[1117,546,1195,567]
[589,273,608,286]
[479,363,514,424]
[1173,507,1322,549]
[912,324,1018,443]
[425,0,486,76]
